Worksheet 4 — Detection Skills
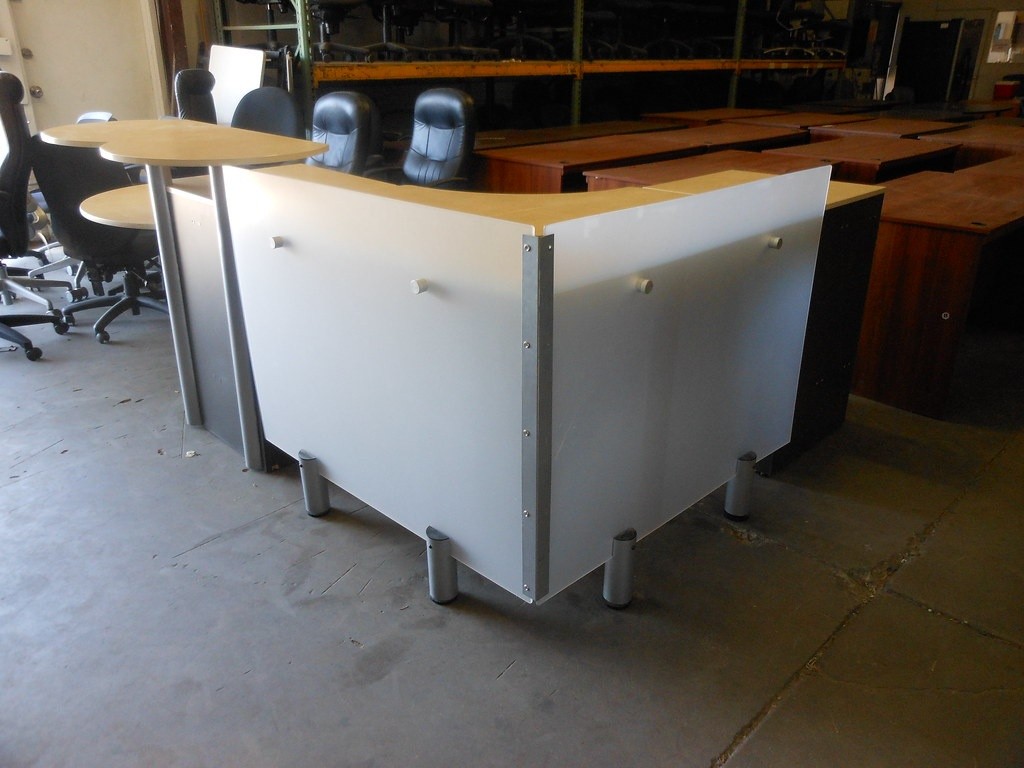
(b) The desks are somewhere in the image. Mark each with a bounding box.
[205,102,1024,618]
[40,116,330,232]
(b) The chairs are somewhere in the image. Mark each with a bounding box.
[230,86,305,140]
[29,134,169,344]
[5,190,50,292]
[363,87,481,191]
[0,72,70,361]
[170,69,218,179]
[305,91,385,180]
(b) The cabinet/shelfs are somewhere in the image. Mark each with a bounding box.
[214,0,906,134]
